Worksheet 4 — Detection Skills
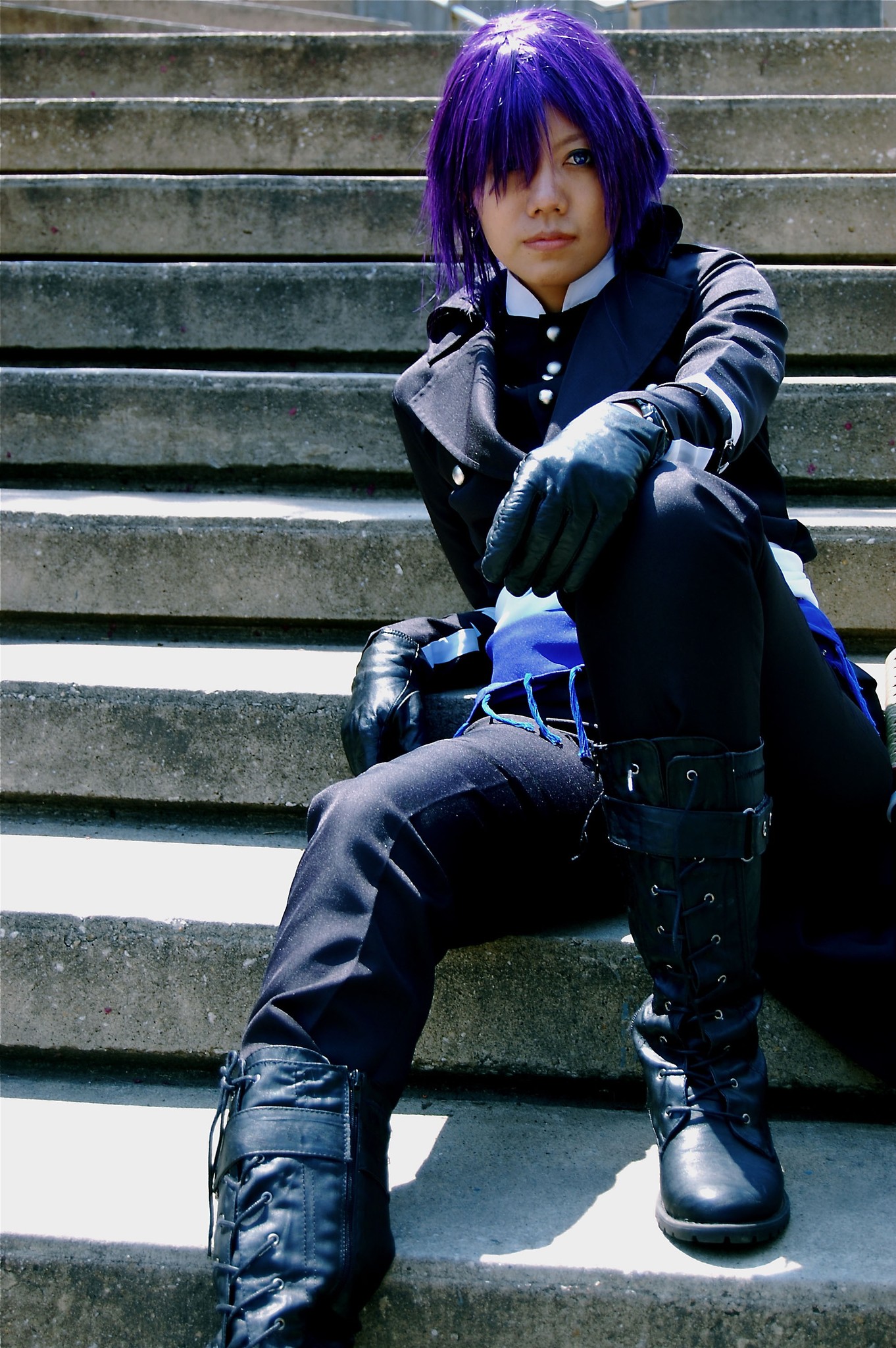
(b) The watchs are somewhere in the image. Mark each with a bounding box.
[631,393,672,452]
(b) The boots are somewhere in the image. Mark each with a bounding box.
[206,1045,394,1348]
[583,737,788,1242]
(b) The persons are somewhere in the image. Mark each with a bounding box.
[195,7,895,1348]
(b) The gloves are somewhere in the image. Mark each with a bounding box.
[483,401,664,597]
[341,628,422,775]
